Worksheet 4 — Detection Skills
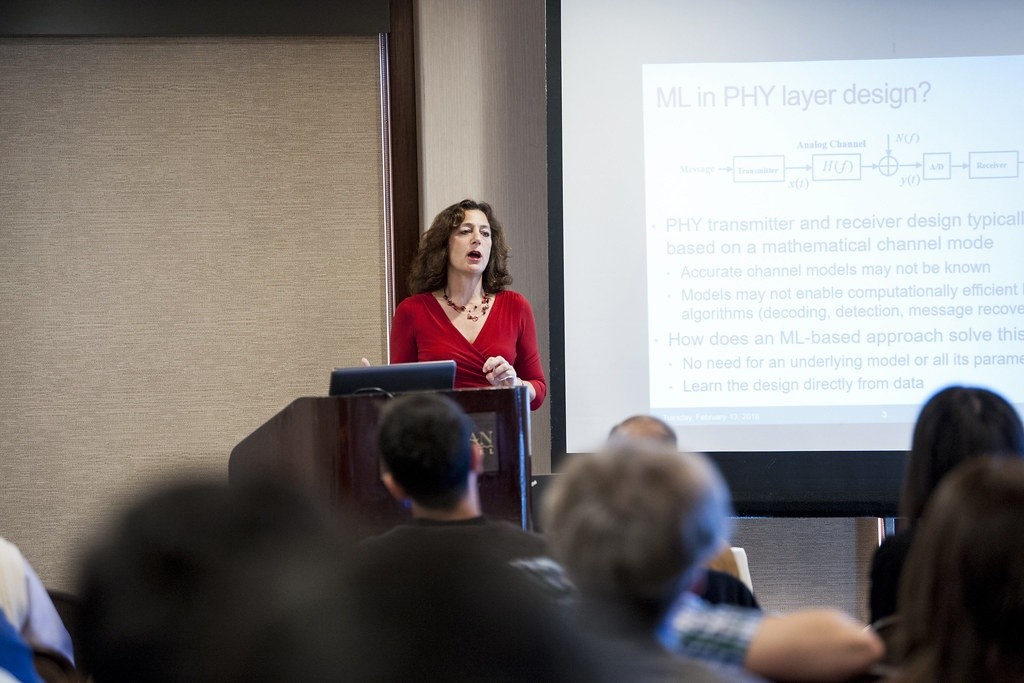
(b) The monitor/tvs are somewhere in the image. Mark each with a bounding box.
[328,360,456,396]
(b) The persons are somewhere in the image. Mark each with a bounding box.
[0,385,1023,682]
[389,199,547,410]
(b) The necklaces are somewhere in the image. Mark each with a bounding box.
[443,286,490,322]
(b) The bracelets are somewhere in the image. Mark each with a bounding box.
[517,377,524,389]
